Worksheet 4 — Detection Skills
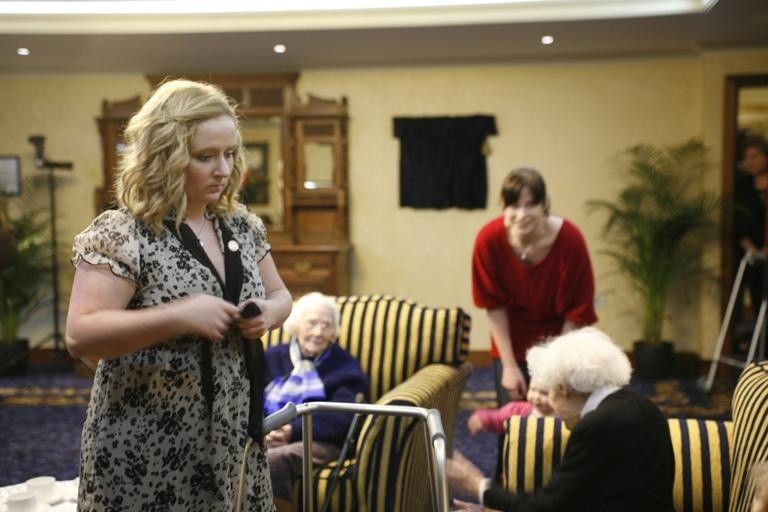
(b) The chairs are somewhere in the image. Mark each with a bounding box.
[502,362,767,512]
[260,293,471,510]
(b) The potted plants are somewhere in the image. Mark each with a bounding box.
[0,169,78,378]
[585,138,721,362]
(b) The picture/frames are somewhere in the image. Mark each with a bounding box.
[0,155,21,194]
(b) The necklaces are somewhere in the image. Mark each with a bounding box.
[508,221,545,262]
[188,216,207,247]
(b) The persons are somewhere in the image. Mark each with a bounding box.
[736,136,768,365]
[472,168,598,471]
[447,327,676,512]
[468,376,565,434]
[261,291,373,512]
[65,76,293,512]
